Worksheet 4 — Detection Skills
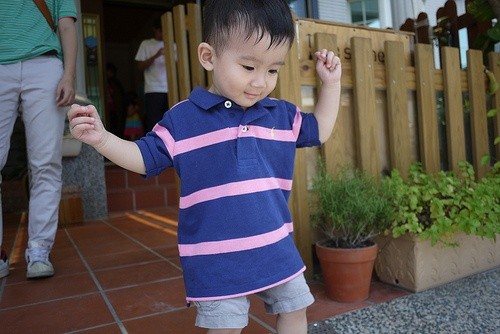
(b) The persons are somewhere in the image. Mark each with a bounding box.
[67,0,342,334]
[0,0,81,278]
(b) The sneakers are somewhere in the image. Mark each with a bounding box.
[25,248,55,279]
[0,250,10,279]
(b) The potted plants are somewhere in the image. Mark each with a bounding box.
[373,156,500,293]
[311,156,393,304]
